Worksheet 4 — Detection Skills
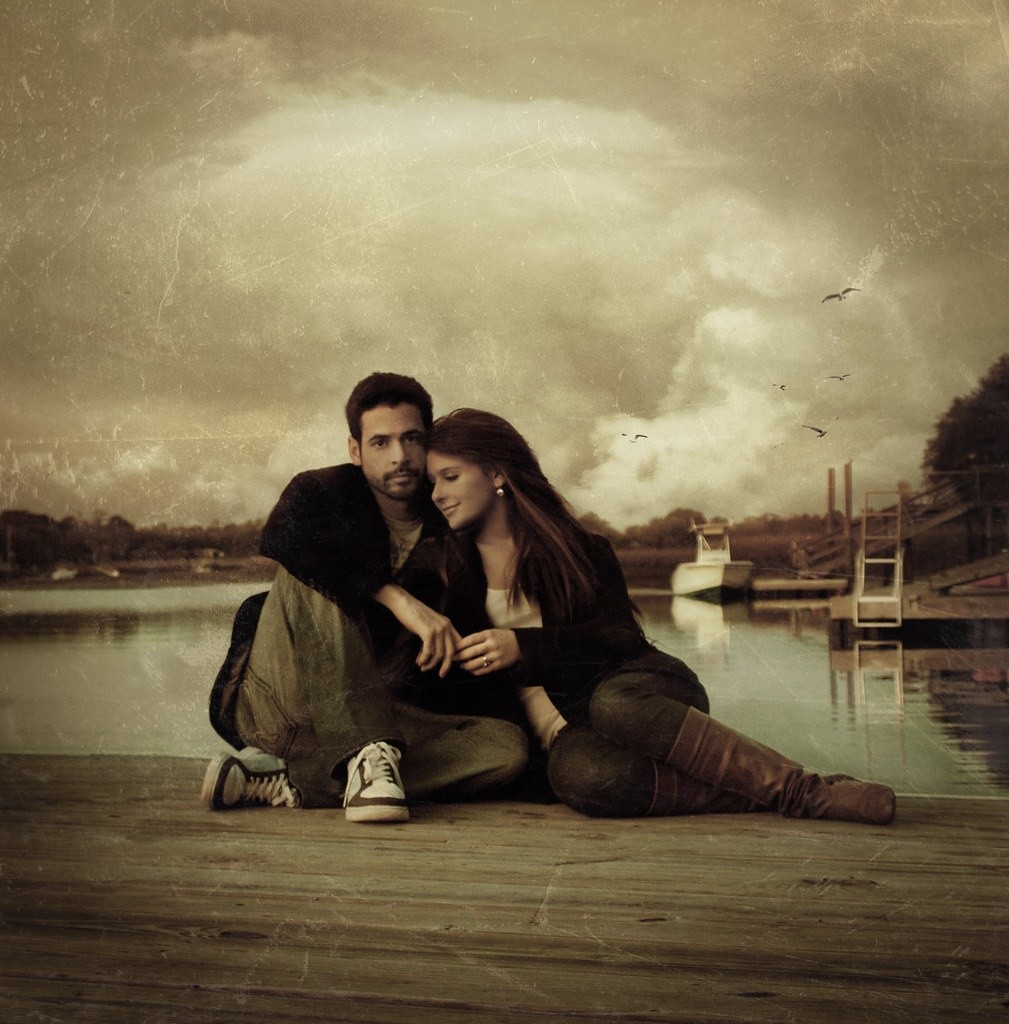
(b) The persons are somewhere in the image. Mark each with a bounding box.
[200,372,897,822]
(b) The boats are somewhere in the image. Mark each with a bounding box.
[671,515,754,606]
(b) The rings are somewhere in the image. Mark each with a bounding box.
[483,656,488,667]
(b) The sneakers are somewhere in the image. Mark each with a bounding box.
[343,742,410,821]
[203,747,300,810]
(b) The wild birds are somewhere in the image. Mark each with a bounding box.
[828,373,851,380]
[772,383,790,390]
[621,433,648,443]
[822,287,863,303]
[801,416,840,438]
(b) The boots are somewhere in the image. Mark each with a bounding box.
[664,706,896,825]
[647,755,861,816]
